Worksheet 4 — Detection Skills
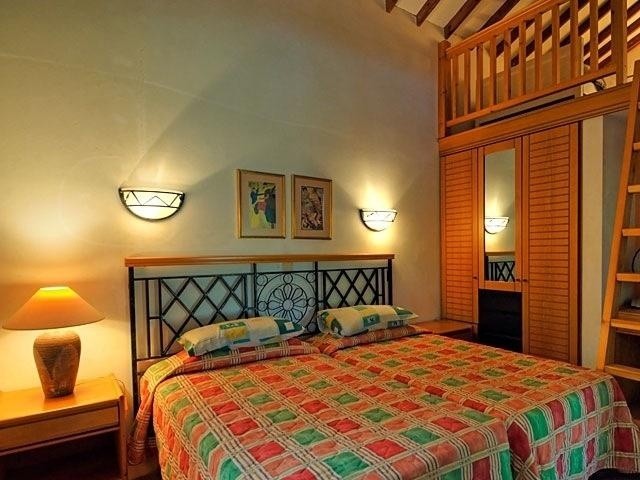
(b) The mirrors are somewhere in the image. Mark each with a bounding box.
[477,139,522,295]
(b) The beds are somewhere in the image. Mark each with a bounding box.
[125,256,640,480]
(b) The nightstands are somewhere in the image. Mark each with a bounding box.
[0,377,126,480]
[413,318,474,342]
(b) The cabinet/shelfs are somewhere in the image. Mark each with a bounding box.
[440,147,476,331]
[522,125,579,367]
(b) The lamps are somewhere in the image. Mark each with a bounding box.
[361,209,397,230]
[122,187,187,221]
[2,288,108,401]
[485,218,510,234]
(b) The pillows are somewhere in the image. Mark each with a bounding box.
[317,304,416,338]
[177,315,303,359]
[306,324,418,356]
[172,339,320,381]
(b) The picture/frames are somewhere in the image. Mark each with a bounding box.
[291,175,334,239]
[235,169,286,240]
